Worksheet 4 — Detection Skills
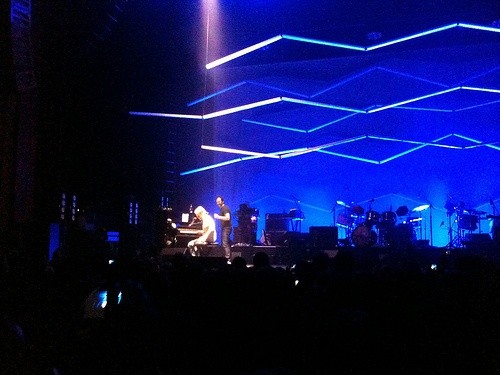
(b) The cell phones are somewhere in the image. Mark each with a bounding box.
[92,289,123,308]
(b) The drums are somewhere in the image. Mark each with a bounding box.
[336,211,397,247]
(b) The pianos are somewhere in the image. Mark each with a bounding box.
[161,223,202,248]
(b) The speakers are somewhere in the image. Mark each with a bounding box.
[265,213,338,248]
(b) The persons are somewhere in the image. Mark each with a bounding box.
[213,197,233,259]
[0,240,500,375]
[188,205,217,258]
